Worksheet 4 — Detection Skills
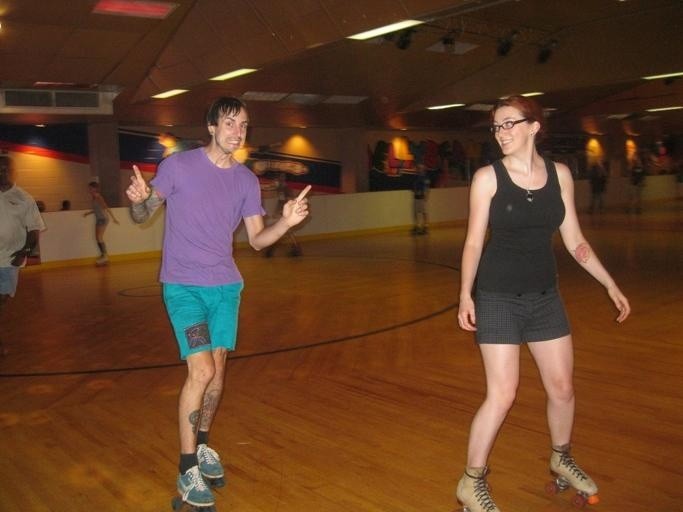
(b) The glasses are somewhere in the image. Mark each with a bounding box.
[490,119,528,133]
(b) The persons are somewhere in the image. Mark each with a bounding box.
[126,96,312,507]
[584,160,607,215]
[623,157,647,215]
[263,172,305,256]
[409,164,430,235]
[60,200,71,211]
[457,93,632,512]
[0,155,46,307]
[82,182,120,267]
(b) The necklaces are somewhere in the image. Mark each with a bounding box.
[525,187,533,203]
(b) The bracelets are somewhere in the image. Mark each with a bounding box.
[24,247,31,253]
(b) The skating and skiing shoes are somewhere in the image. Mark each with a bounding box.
[195,443,224,488]
[171,462,217,511]
[95,251,112,266]
[411,224,428,236]
[546,443,599,507]
[455,468,498,512]
